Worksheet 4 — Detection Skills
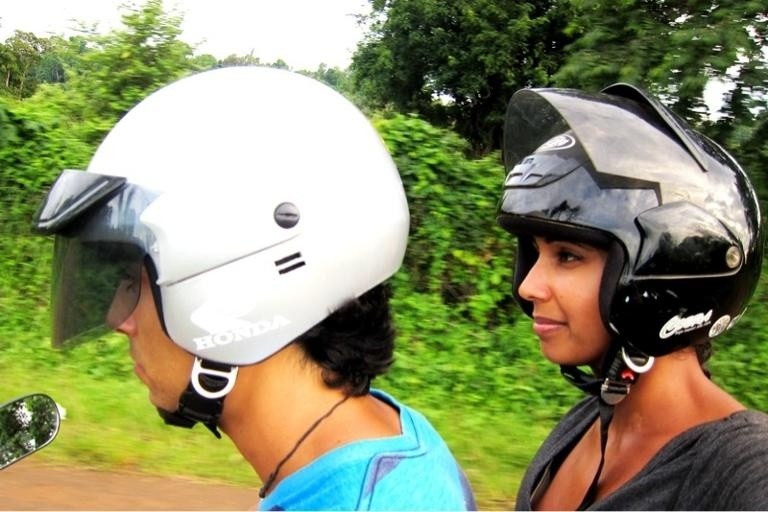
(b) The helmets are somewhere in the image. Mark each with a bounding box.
[497,81,764,409]
[26,64,415,442]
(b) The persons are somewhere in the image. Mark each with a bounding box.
[30,65,477,512]
[496,81,768,509]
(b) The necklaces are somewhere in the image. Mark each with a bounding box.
[257,395,351,499]
